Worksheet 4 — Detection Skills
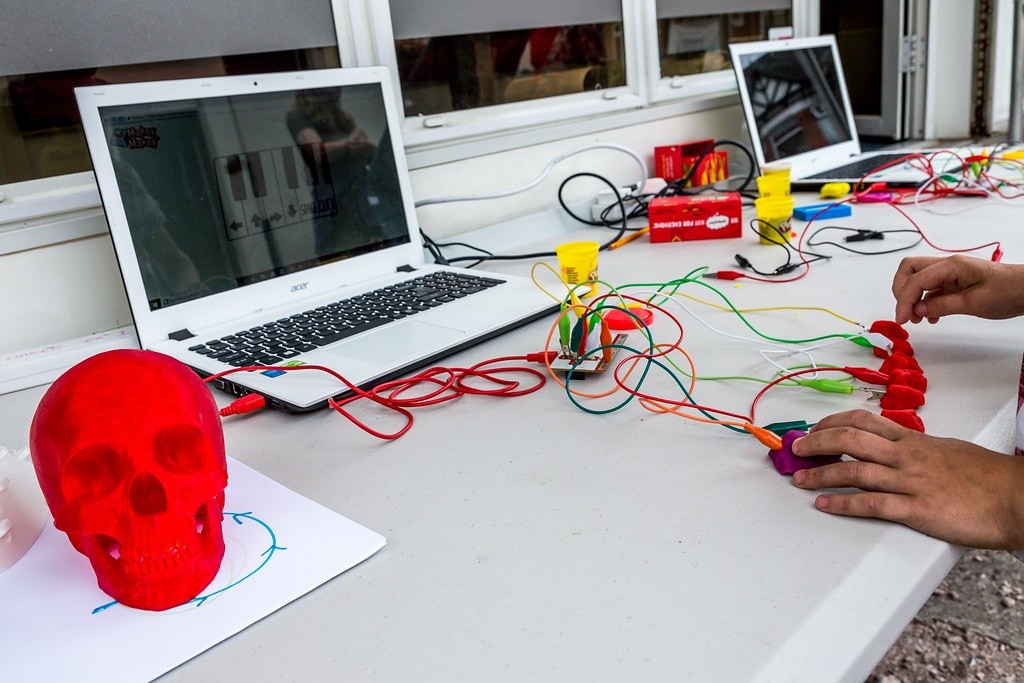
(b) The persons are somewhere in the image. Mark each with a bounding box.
[283,86,374,262]
[791,254,1024,561]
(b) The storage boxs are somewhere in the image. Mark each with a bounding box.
[656,138,729,187]
[648,190,743,242]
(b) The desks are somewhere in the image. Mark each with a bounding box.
[0,140,1024,683]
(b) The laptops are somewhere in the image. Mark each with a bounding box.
[728,34,994,188]
[74,66,592,415]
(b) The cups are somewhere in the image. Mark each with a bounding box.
[556,241,599,299]
[754,197,793,245]
[756,167,791,198]
